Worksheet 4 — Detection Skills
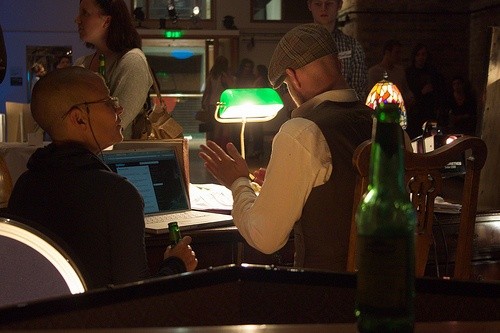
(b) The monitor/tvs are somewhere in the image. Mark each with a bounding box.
[136,40,206,95]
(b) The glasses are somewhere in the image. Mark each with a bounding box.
[61,96,120,119]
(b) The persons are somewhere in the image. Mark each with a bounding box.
[202,55,268,161]
[199,22,413,272]
[366,39,478,136]
[6,66,197,289]
[308,0,368,100]
[72,0,152,140]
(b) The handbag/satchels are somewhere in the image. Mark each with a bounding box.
[195,106,210,123]
[106,45,184,141]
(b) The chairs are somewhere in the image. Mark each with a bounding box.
[0,216,88,306]
[347,137,489,281]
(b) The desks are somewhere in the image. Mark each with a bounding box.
[145,210,500,277]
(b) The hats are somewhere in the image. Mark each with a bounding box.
[268,24,338,90]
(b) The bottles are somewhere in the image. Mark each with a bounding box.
[355,103,416,333]
[168,221,181,249]
[98,54,105,77]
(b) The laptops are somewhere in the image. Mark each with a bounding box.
[98,148,238,236]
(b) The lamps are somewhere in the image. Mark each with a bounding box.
[167,0,177,20]
[368,72,407,128]
[215,87,284,159]
